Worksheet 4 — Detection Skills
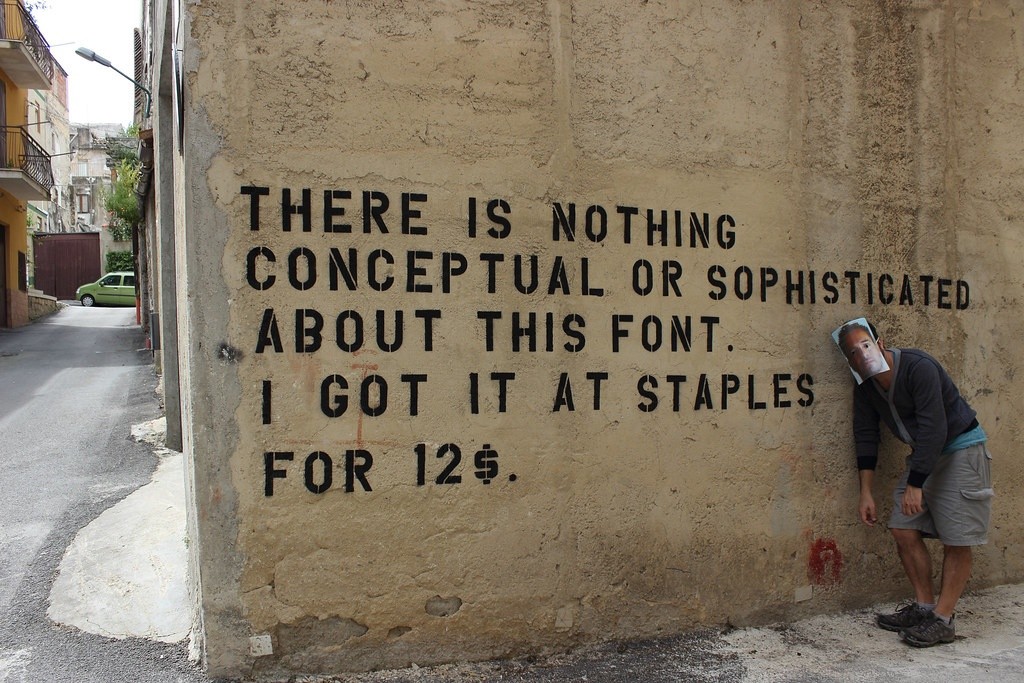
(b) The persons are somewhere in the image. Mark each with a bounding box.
[840,317,994,647]
[838,322,882,381]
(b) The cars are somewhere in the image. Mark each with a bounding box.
[75,272,137,308]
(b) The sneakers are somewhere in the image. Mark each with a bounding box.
[876,605,957,648]
